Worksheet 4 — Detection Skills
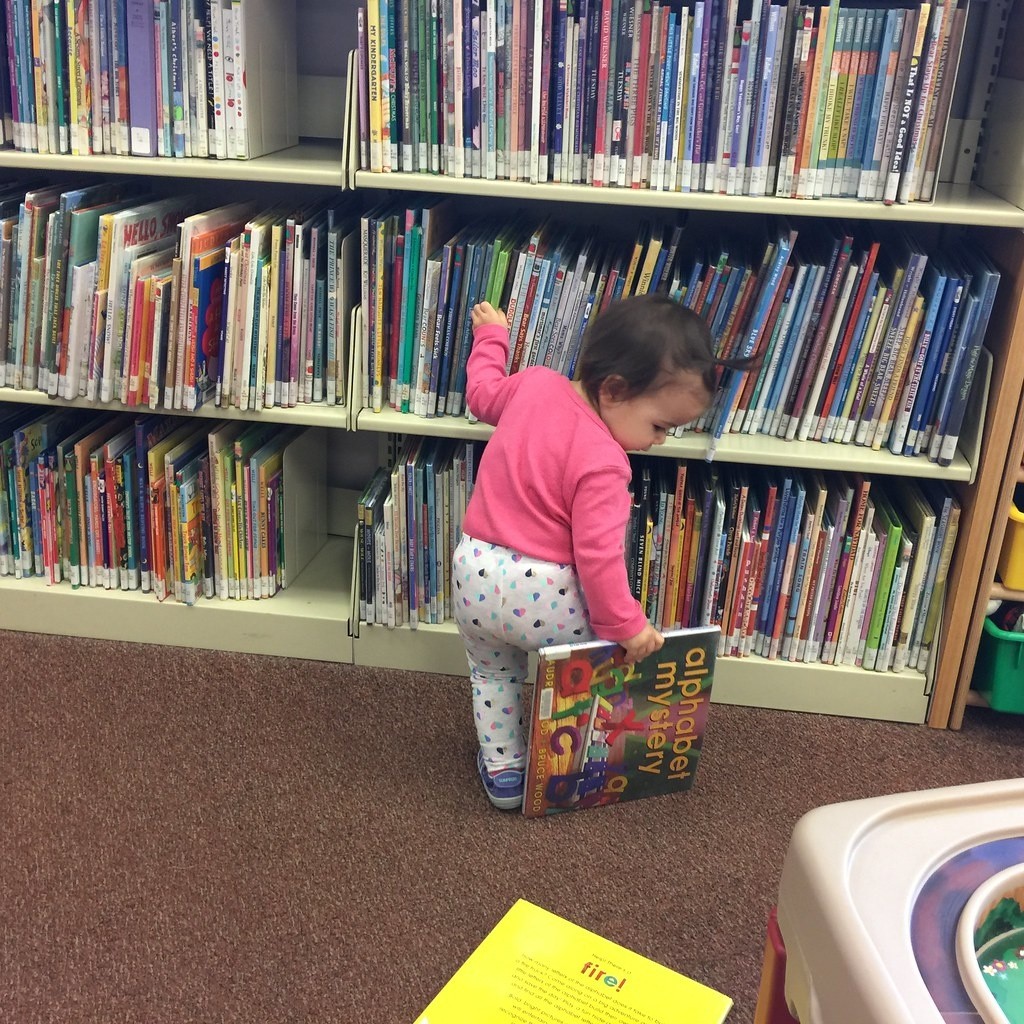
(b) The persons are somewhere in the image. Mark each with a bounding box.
[450,295,766,812]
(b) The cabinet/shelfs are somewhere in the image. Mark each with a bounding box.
[0,1,1024,730]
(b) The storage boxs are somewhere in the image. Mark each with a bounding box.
[982,613,1021,713]
[976,497,1023,601]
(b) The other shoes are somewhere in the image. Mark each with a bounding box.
[476,746,527,809]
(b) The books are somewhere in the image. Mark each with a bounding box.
[0,0,1011,676]
[521,624,720,818]
[411,897,734,1024]
